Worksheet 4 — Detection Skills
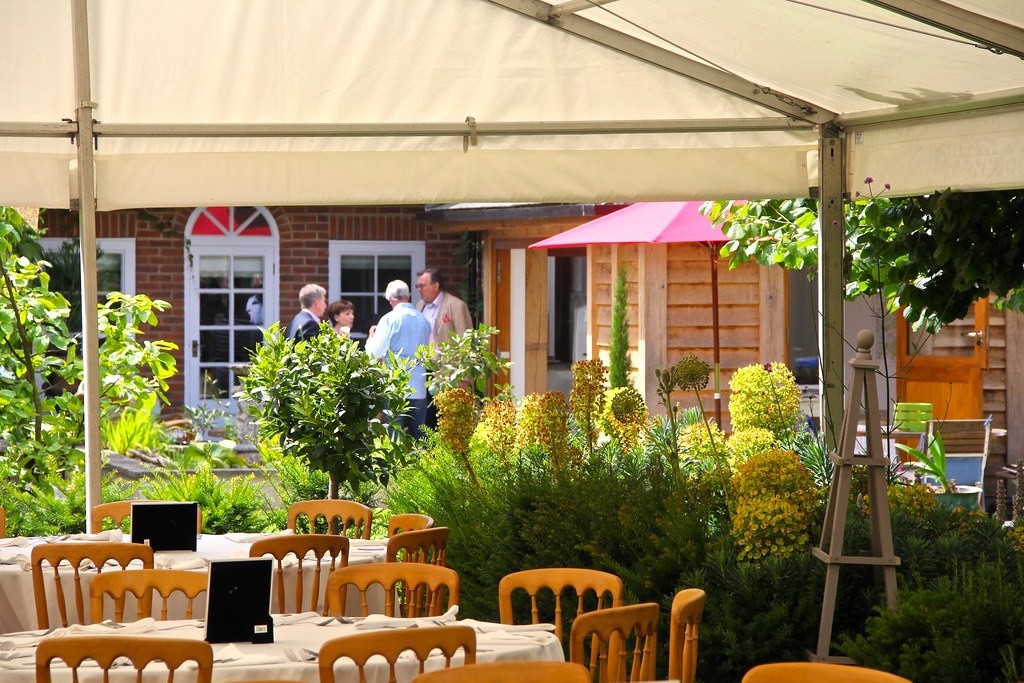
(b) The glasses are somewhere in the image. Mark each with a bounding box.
[416,283,432,290]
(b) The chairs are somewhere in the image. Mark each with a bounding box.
[30,541,153,630]
[570,604,658,683]
[250,534,350,618]
[288,500,373,539]
[89,501,201,535]
[88,569,208,622]
[35,638,212,683]
[326,563,460,619]
[387,527,451,616]
[387,514,433,563]
[497,568,622,683]
[666,588,706,683]
[319,626,478,681]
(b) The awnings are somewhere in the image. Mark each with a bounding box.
[0,1,1024,538]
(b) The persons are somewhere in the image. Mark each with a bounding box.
[365,280,432,438]
[286,284,326,342]
[412,269,473,424]
[246,277,264,325]
[327,300,354,335]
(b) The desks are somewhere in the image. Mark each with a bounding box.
[0,614,564,683]
[0,534,401,618]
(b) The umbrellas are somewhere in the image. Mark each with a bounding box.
[528,199,777,428]
